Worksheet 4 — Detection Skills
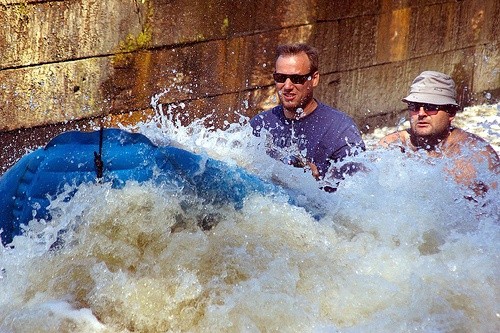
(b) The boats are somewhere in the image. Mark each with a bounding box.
[0,126,326,247]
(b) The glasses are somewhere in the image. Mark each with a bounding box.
[273,71,314,83]
[407,102,448,115]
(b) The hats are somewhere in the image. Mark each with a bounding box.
[402,71,458,105]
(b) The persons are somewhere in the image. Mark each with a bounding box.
[374,71,500,201]
[250,42,366,193]
